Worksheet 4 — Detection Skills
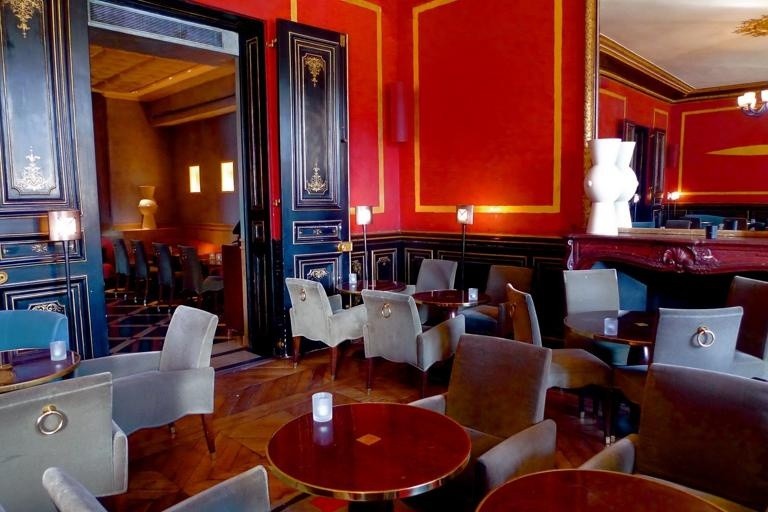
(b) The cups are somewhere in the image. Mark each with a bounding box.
[723,219,738,230]
[407,285,415,296]
[751,219,755,231]
[604,318,618,335]
[706,225,717,240]
[313,393,332,421]
[210,254,215,259]
[51,341,66,360]
[349,273,357,284]
[216,253,222,262]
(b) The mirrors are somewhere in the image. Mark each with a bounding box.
[582,0,768,233]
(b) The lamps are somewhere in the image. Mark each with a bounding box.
[737,87,768,116]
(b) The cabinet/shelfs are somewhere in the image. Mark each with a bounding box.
[349,230,571,347]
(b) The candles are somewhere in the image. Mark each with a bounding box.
[348,272,357,285]
[605,316,619,335]
[311,392,333,423]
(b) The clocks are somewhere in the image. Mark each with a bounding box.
[458,205,473,225]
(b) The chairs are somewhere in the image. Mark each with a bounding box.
[504,280,614,447]
[111,237,136,298]
[1,371,129,512]
[1,309,70,366]
[559,267,652,428]
[49,340,68,361]
[40,464,273,512]
[75,305,220,453]
[359,288,466,397]
[408,334,553,510]
[175,245,225,320]
[725,275,768,361]
[461,264,533,335]
[577,363,767,511]
[151,242,196,312]
[128,239,159,303]
[410,258,458,325]
[284,276,368,379]
